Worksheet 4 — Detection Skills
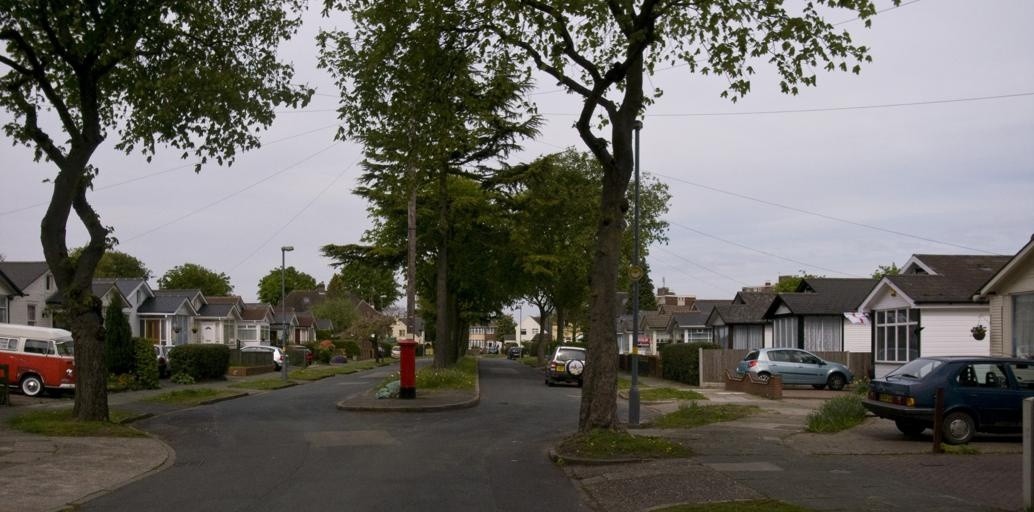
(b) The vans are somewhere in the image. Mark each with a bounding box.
[0,324,77,397]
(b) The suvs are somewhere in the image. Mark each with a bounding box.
[545,346,586,387]
[507,346,522,361]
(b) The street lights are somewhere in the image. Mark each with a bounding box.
[629,120,646,428]
[279,246,294,379]
[516,306,523,348]
[379,294,389,311]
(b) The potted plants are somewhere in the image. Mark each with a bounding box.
[971,325,988,340]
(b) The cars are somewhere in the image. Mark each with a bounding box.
[240,346,284,371]
[864,355,1033,446]
[391,346,402,359]
[488,346,499,355]
[154,344,170,370]
[737,348,854,390]
[289,346,313,365]
